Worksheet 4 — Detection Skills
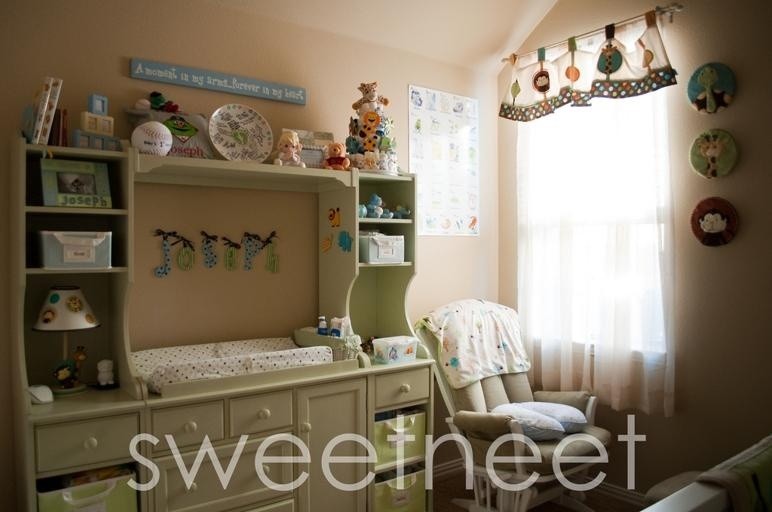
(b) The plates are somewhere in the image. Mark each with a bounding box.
[206,101,274,164]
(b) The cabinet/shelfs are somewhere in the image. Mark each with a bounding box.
[8,130,439,512]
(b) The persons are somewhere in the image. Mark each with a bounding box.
[273,129,306,168]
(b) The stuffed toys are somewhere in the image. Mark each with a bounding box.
[323,82,397,172]
[359,192,410,219]
[52,345,115,389]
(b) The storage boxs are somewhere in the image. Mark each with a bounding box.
[36,469,139,511]
[372,333,417,364]
[358,232,407,267]
[32,285,103,399]
[39,227,113,271]
[371,408,427,470]
[374,466,426,512]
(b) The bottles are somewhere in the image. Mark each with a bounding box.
[316,316,327,335]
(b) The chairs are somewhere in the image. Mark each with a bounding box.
[413,296,613,512]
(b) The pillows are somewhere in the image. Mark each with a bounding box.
[526,398,586,433]
[490,402,562,443]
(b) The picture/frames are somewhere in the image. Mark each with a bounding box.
[39,156,114,210]
